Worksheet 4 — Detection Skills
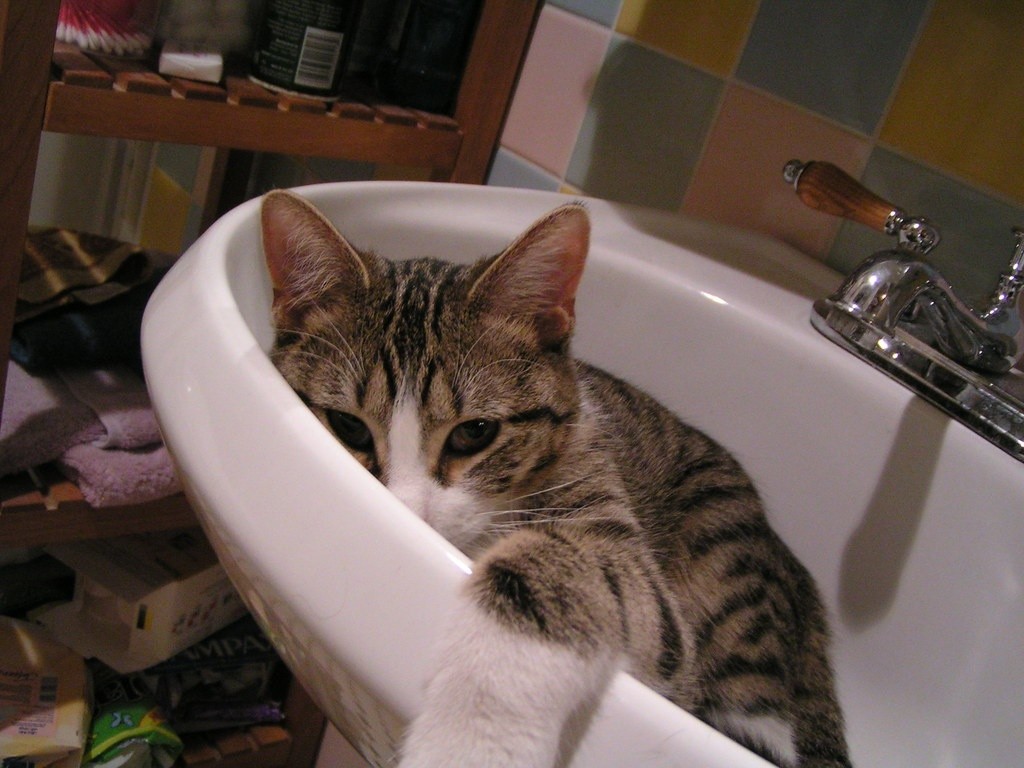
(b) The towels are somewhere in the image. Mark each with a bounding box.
[0,358,185,509]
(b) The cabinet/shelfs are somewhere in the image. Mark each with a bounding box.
[0,0,548,768]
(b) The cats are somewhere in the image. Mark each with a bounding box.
[258,189,854,768]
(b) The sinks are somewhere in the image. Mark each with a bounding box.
[139,180,1024,768]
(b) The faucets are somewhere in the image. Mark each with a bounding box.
[810,227,1024,466]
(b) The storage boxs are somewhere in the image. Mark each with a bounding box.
[34,530,247,675]
